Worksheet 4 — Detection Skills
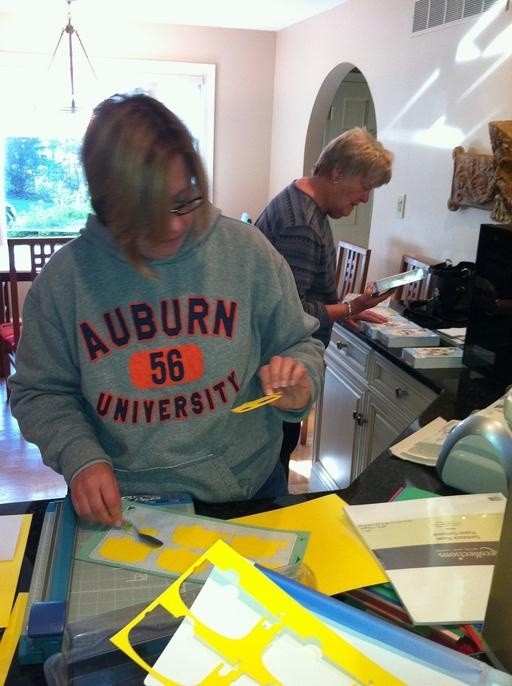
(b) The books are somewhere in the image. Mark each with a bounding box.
[369,266,427,293]
[359,306,465,370]
[339,489,506,626]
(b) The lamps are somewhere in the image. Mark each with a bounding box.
[34,0,109,119]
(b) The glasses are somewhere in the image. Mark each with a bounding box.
[168,177,205,216]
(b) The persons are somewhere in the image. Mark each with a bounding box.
[9,93,329,532]
[249,127,396,473]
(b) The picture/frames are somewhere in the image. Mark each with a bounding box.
[0,49,216,246]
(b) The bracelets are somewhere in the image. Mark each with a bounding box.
[344,301,353,321]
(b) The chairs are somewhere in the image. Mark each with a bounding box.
[393,253,433,297]
[1,236,73,403]
[333,239,372,294]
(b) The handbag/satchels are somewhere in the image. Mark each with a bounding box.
[430,259,499,322]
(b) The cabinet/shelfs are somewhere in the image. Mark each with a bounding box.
[305,301,483,496]
